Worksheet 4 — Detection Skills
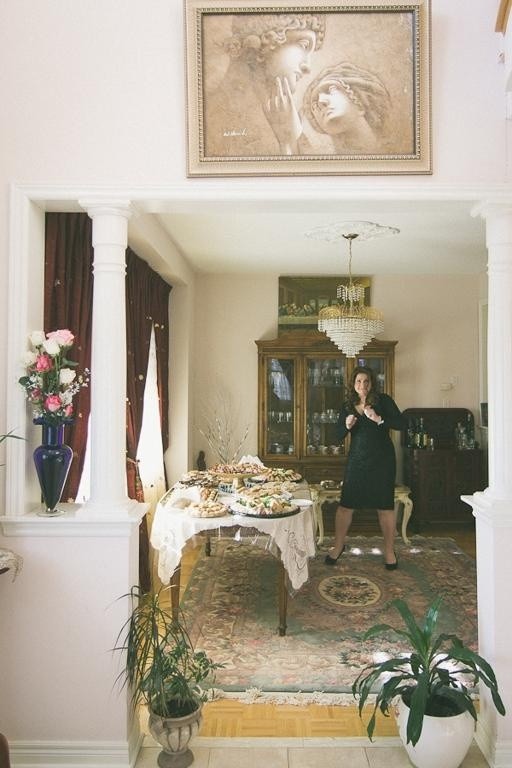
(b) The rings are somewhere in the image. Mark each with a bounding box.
[368,415,371,417]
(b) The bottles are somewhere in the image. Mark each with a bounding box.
[416,415,428,449]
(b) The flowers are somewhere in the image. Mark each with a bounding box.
[18,329,93,420]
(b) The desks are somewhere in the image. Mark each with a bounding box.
[310,483,414,547]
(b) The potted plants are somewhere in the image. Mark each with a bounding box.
[352,592,506,767]
[103,567,228,768]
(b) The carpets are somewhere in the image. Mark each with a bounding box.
[139,535,477,707]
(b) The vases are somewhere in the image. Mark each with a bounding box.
[32,418,74,517]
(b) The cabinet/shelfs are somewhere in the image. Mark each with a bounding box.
[255,328,398,526]
[401,408,484,533]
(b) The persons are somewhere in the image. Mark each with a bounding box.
[256,60,393,155]
[324,365,406,571]
[207,14,326,157]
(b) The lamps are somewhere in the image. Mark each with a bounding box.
[304,221,400,358]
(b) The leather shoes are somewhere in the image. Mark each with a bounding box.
[385,552,398,570]
[326,545,345,565]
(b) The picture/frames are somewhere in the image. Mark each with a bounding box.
[183,1,433,178]
